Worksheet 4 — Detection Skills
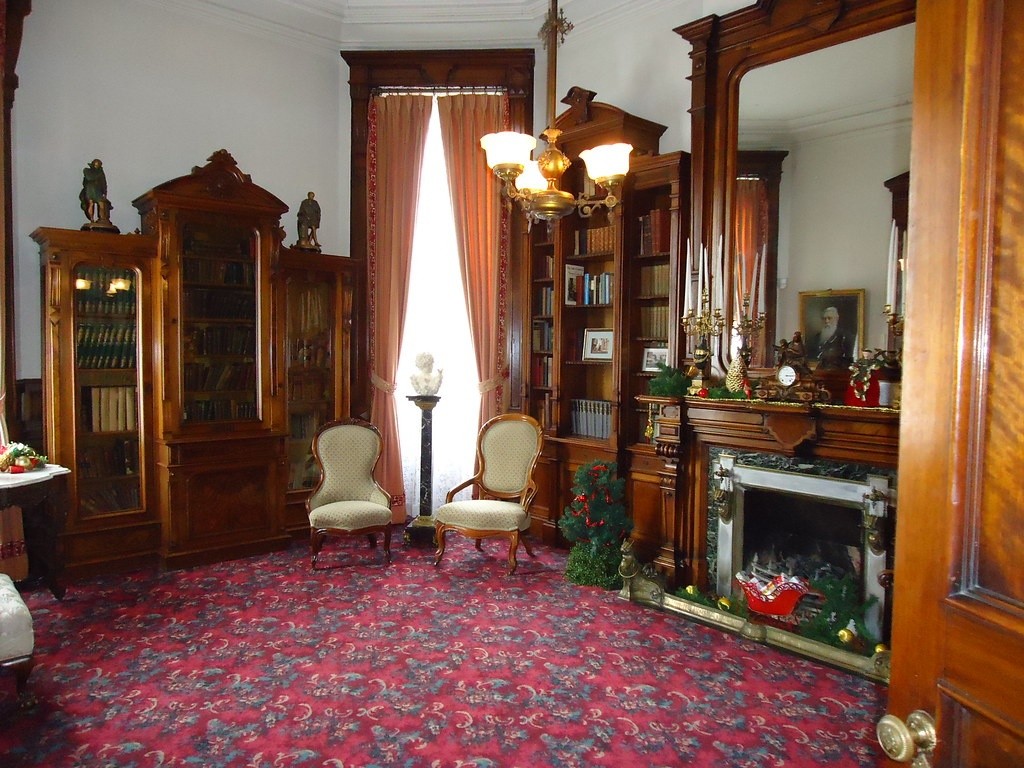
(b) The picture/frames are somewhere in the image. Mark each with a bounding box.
[583,327,614,363]
[635,341,668,372]
[798,287,865,361]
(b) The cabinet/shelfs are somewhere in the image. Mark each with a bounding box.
[183,253,256,421]
[520,85,693,577]
[74,314,144,517]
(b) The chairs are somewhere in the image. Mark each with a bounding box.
[305,417,400,570]
[431,413,544,575]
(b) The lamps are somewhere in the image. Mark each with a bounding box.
[480,0,634,235]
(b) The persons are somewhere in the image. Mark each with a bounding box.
[297,192,323,246]
[410,352,442,395]
[83,159,107,222]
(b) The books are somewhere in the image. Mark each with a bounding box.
[534,356,553,387]
[637,208,668,254]
[641,306,669,337]
[77,269,136,368]
[544,256,554,278]
[640,264,670,295]
[574,224,616,256]
[185,229,259,421]
[569,399,612,438]
[566,264,614,305]
[533,319,554,351]
[538,286,554,315]
[92,386,136,431]
[537,393,550,428]
[649,402,661,443]
[290,292,331,486]
[79,440,139,513]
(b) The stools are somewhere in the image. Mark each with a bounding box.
[0,573,34,708]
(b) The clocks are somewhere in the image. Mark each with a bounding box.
[757,338,830,404]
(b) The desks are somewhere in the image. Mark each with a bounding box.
[0,461,72,602]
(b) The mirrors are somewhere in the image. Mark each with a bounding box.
[731,21,915,406]
